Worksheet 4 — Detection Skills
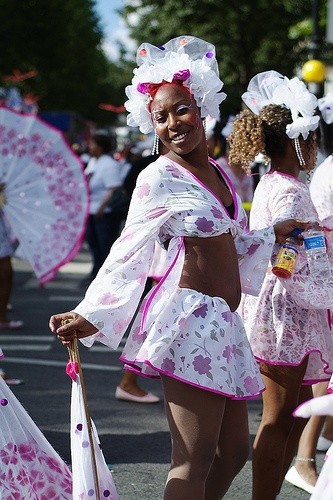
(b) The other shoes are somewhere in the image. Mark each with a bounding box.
[0,320,23,330]
[81,274,93,287]
[115,386,161,403]
[315,436,333,450]
[285,465,322,495]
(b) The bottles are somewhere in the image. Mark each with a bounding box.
[303,217,331,279]
[272,228,301,278]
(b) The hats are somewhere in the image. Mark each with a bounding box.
[91,129,117,150]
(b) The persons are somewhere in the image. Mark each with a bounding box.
[73,128,170,290]
[0,181,24,331]
[208,61,333,500]
[49,35,311,500]
